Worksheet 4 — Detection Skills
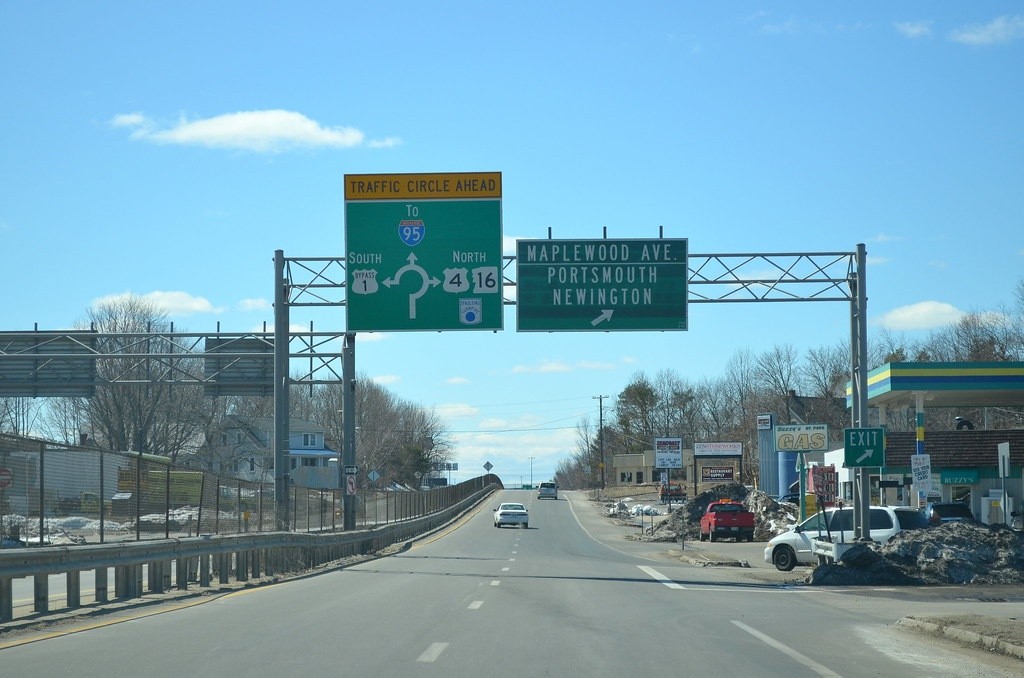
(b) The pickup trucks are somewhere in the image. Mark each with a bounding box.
[699,499,755,542]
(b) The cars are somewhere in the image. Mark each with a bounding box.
[778,493,834,508]
[535,481,559,500]
[659,484,687,500]
[493,503,529,529]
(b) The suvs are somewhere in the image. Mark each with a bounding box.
[922,502,974,523]
[764,506,929,571]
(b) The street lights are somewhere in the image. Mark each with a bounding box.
[599,408,614,490]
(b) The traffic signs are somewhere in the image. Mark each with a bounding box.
[515,237,689,332]
[844,427,885,469]
[343,172,506,333]
[345,466,358,475]
